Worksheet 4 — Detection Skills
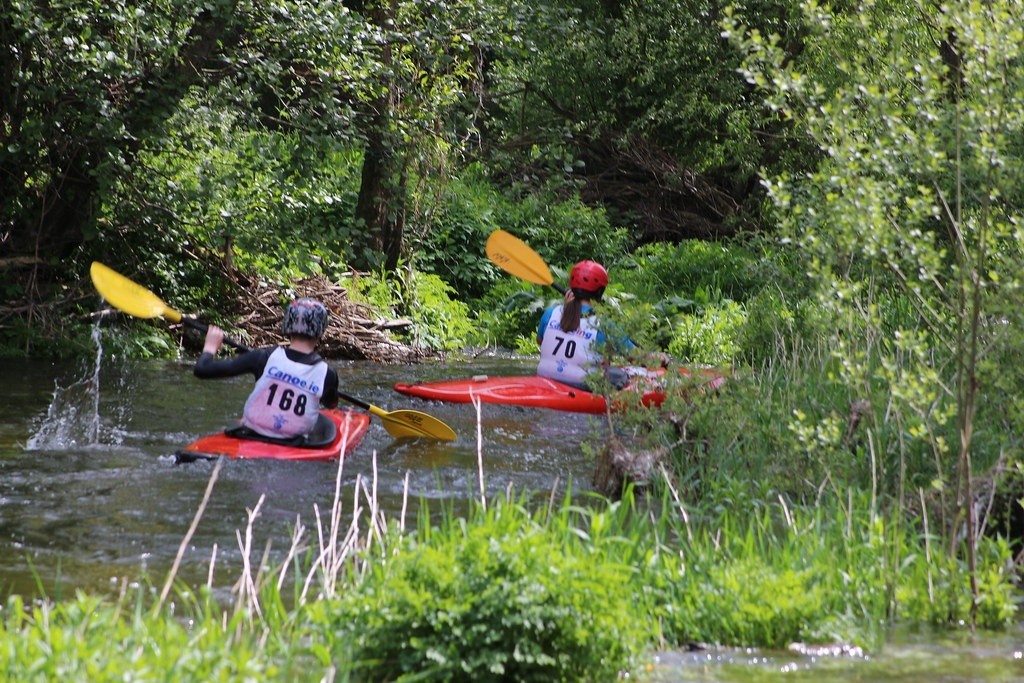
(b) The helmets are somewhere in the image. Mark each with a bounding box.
[569,260,608,293]
[282,297,329,337]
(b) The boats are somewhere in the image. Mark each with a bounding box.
[176,409,372,463]
[394,366,729,416]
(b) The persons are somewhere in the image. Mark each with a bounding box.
[536,261,669,395]
[193,299,339,444]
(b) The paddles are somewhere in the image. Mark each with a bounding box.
[89,258,460,444]
[484,228,679,372]
